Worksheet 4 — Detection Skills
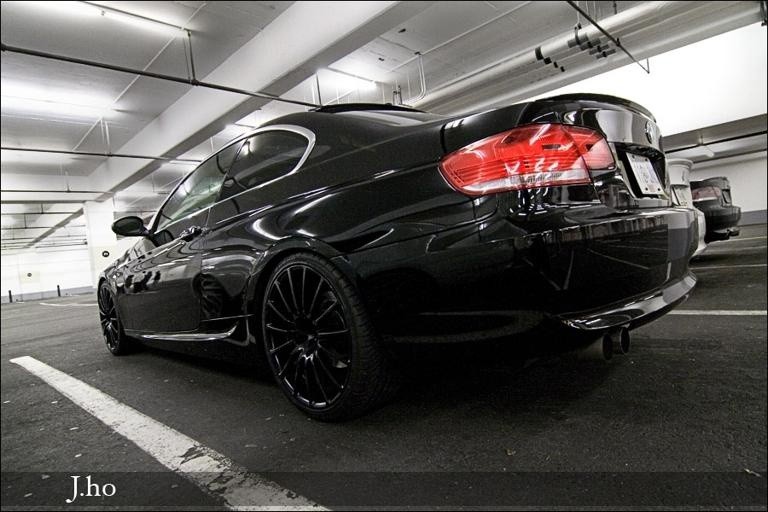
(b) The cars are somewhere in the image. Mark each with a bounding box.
[94,88,712,423]
[656,146,748,258]
